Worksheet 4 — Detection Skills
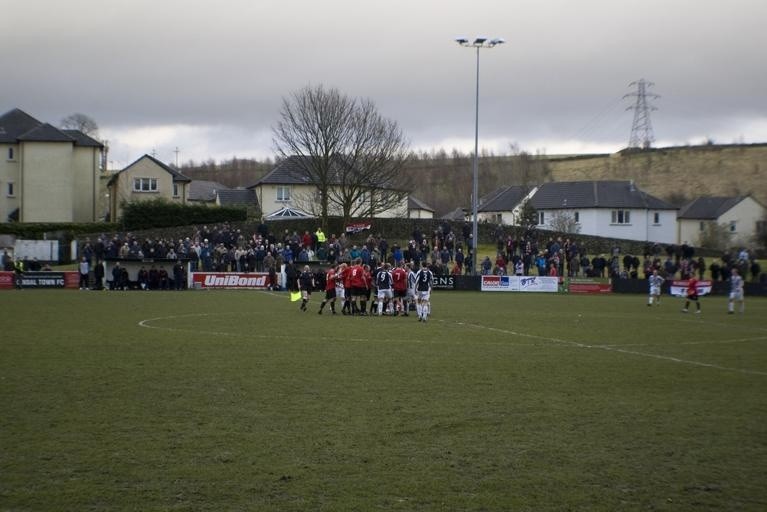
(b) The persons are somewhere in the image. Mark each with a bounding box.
[441,223,471,242]
[647,269,664,306]
[479,222,762,283]
[297,264,315,311]
[728,268,745,314]
[0,228,473,292]
[317,258,434,322]
[681,272,701,314]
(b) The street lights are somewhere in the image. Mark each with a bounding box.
[455,35,504,272]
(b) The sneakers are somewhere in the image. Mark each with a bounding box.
[695,310,701,314]
[319,307,410,315]
[682,308,688,313]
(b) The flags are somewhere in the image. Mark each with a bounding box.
[290,291,301,301]
